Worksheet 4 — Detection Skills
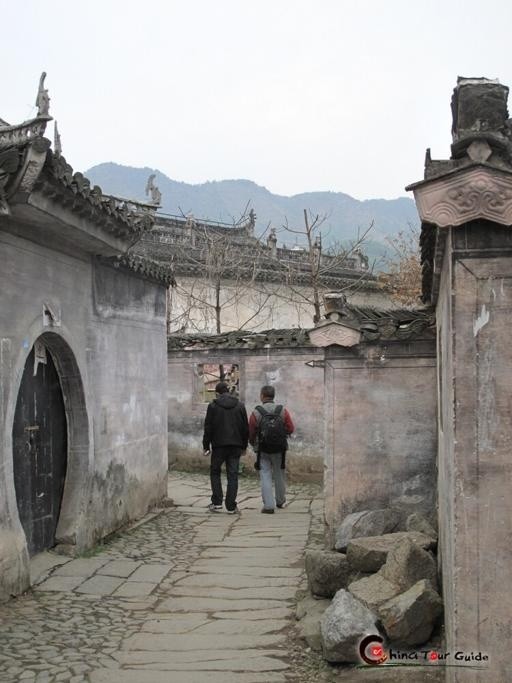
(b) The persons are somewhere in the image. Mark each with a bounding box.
[202,382,248,513]
[248,385,295,513]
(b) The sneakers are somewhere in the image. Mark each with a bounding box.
[277,498,286,508]
[225,506,240,515]
[260,508,274,513]
[208,503,223,513]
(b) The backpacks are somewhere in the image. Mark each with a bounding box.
[254,404,288,454]
